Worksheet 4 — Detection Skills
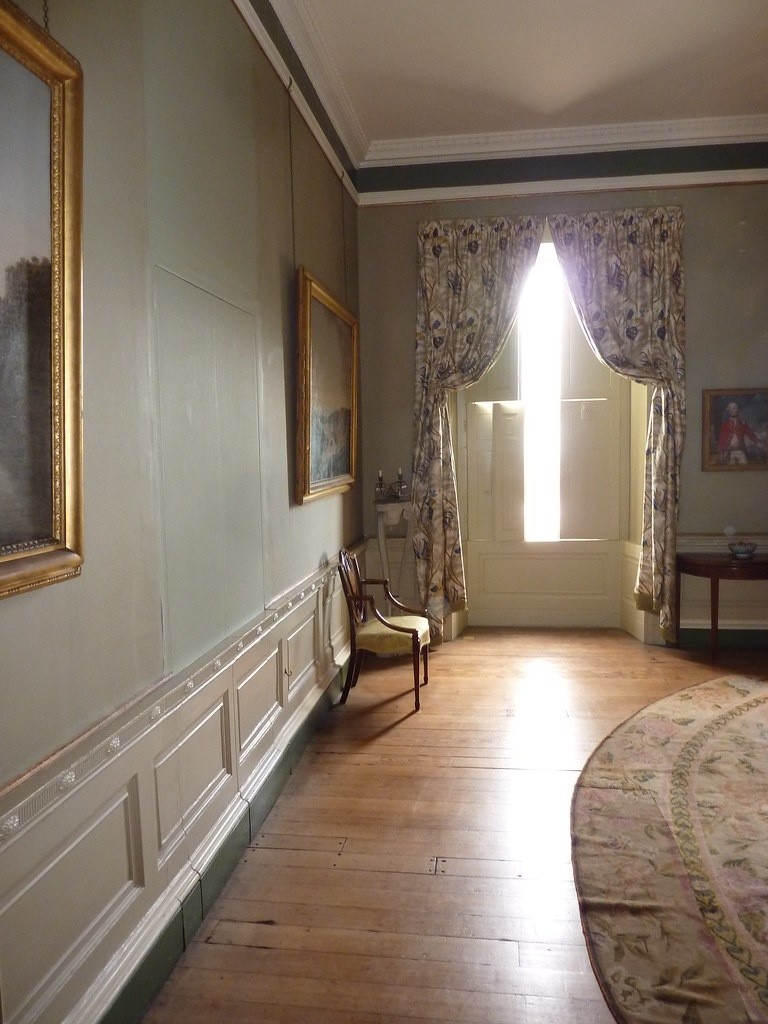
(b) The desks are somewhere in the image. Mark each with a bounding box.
[676,552,768,664]
[374,499,413,617]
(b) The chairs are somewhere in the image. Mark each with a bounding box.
[338,548,431,711]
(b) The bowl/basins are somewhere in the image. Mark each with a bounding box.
[729,543,757,558]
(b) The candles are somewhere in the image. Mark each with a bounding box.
[398,467,402,475]
[378,470,382,477]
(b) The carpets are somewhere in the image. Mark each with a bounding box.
[571,673,768,1024]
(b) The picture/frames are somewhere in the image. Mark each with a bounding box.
[701,388,768,471]
[0,0,86,600]
[294,266,360,505]
